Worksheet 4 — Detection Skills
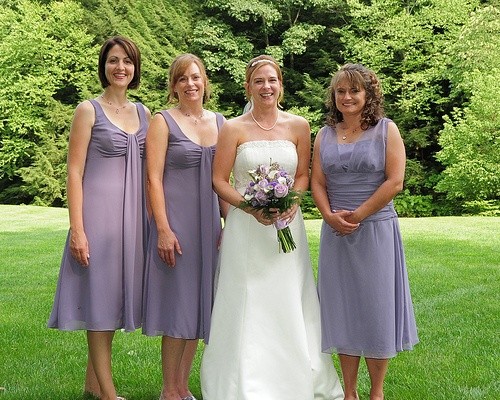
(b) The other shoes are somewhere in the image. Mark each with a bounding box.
[116,396,126,400]
[181,395,197,400]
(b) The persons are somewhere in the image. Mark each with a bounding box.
[200,55,346,400]
[310,64,419,400]
[47,36,152,400]
[141,54,230,400]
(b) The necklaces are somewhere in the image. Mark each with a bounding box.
[178,104,205,126]
[99,93,131,114]
[341,117,362,140]
[250,108,281,131]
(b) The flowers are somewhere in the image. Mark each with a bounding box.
[234,156,314,254]
